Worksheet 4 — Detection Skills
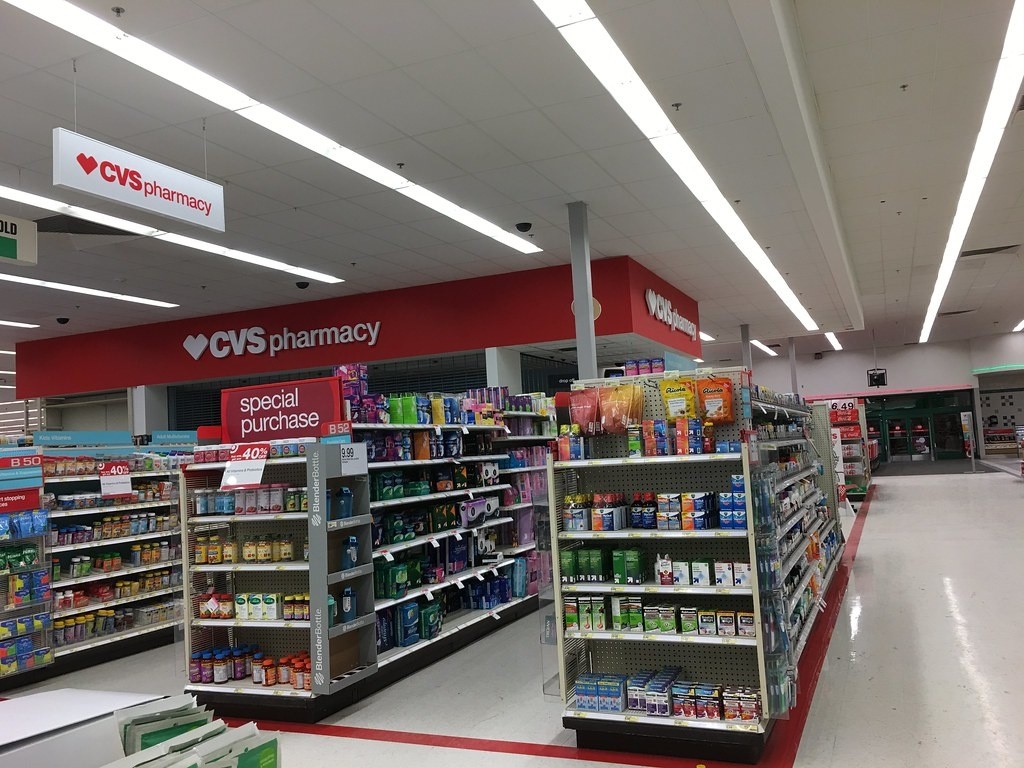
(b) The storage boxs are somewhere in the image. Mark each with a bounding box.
[557,418,762,724]
[829,409,866,493]
[0,570,52,678]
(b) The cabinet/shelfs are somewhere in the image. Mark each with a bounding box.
[44,469,185,658]
[0,530,56,680]
[489,410,559,616]
[751,399,841,667]
[828,421,867,495]
[547,453,766,735]
[178,443,379,700]
[984,425,1024,458]
[350,421,516,668]
